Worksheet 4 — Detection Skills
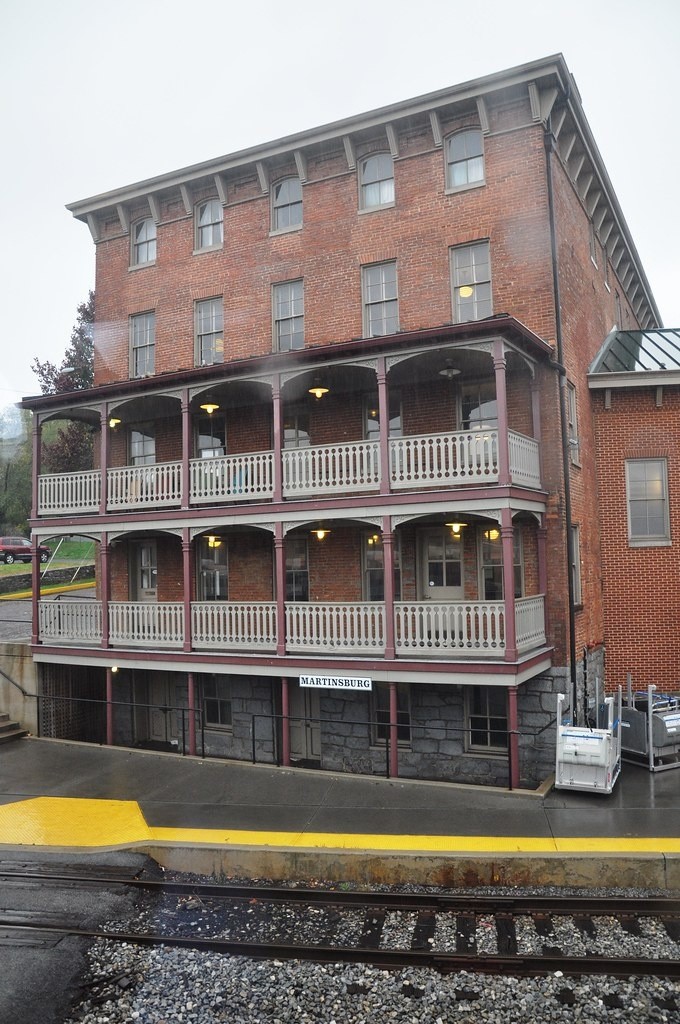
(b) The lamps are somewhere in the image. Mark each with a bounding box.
[373,534,378,542]
[202,535,221,547]
[453,532,461,538]
[208,540,222,547]
[308,385,329,399]
[485,529,500,540]
[310,528,332,539]
[459,286,473,298]
[368,408,379,417]
[445,520,468,532]
[367,538,373,544]
[199,401,220,414]
[437,358,462,378]
[111,667,117,673]
[109,416,122,428]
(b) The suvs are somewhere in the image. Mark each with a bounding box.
[0,535,53,564]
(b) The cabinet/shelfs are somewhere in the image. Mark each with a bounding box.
[554,684,623,795]
[599,671,680,772]
